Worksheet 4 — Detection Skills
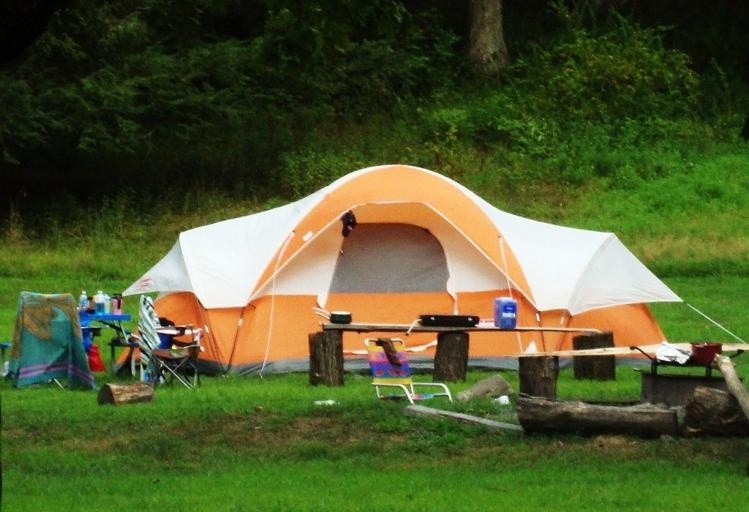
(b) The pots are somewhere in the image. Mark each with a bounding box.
[314,311,352,324]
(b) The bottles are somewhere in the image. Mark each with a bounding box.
[494,298,515,328]
[79,290,121,314]
[185,327,192,342]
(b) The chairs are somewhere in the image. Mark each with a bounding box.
[150,323,200,391]
[365,339,453,404]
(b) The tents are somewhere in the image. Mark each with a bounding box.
[111,163,685,379]
